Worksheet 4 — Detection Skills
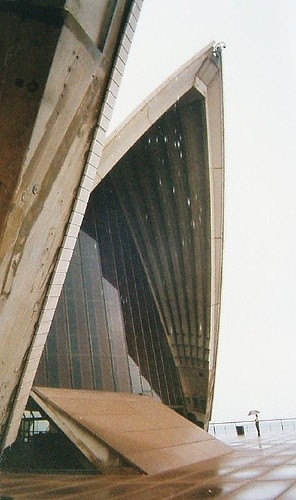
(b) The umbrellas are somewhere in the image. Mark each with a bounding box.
[247,410,260,416]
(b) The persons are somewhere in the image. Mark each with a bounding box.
[255,414,260,437]
